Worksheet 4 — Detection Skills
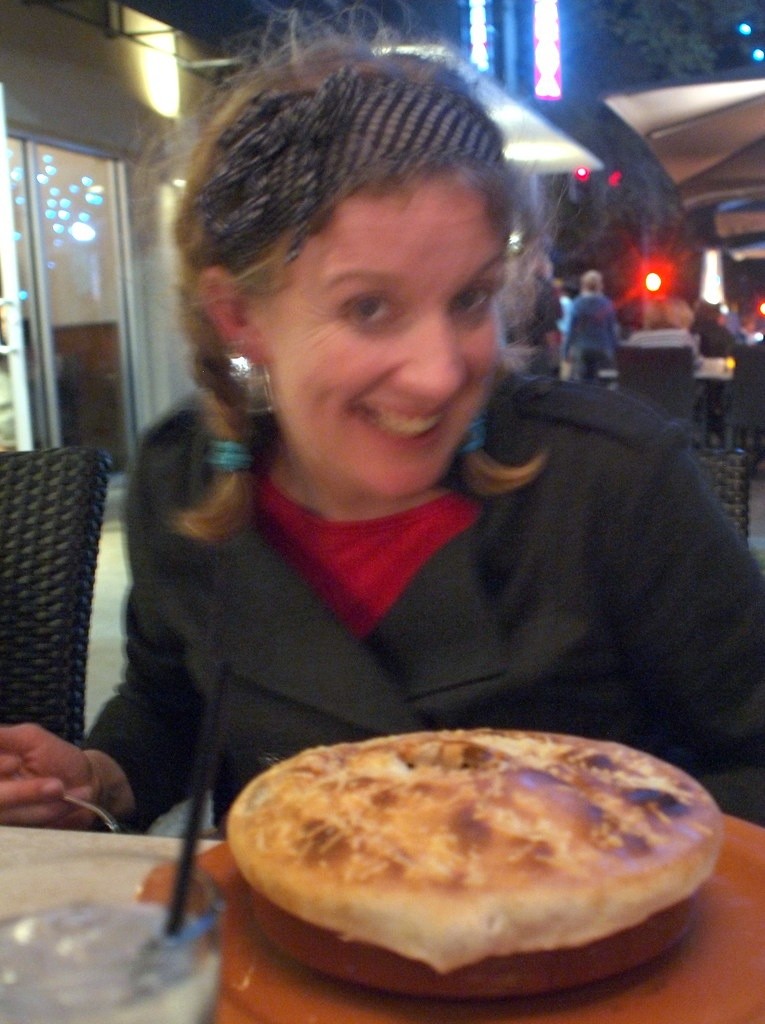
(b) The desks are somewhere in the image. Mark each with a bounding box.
[0,825,222,1024]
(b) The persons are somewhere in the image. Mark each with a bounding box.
[500,256,745,394]
[0,47,765,824]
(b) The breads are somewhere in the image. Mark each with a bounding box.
[228,727,722,1000]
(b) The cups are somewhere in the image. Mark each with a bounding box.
[2,854,225,1024]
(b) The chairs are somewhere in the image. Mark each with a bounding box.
[0,444,114,744]
[612,342,707,450]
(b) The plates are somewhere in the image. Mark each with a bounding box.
[136,802,764,1023]
